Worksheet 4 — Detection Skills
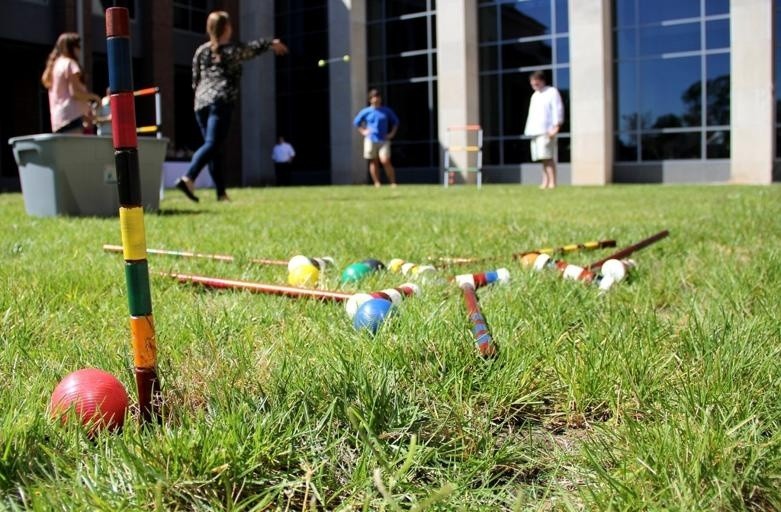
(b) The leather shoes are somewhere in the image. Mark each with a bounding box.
[175,179,199,203]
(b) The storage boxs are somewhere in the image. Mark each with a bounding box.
[7,133,169,219]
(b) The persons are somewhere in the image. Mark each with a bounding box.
[353,90,399,186]
[40,32,102,134]
[175,11,288,201]
[271,136,296,184]
[523,71,565,188]
